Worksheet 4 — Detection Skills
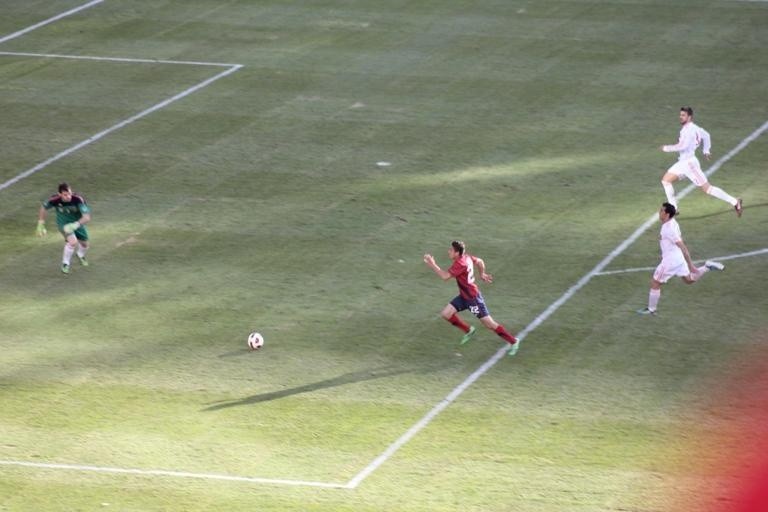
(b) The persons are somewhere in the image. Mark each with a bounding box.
[637,203,725,316]
[35,182,90,274]
[658,105,743,217]
[423,239,520,356]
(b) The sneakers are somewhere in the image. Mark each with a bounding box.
[460,326,478,347]
[62,261,69,273]
[637,308,657,316]
[735,198,743,216]
[508,338,520,356]
[75,253,88,267]
[705,260,725,273]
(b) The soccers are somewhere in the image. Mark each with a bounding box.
[247,332,263,350]
